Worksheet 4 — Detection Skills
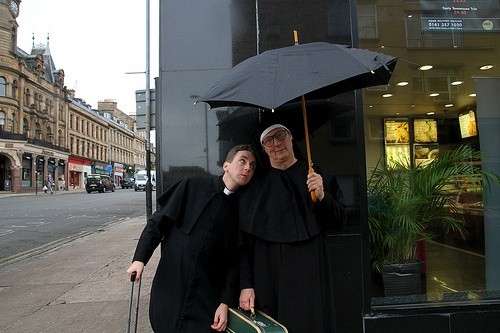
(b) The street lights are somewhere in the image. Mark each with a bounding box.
[26,154,45,195]
[50,159,64,194]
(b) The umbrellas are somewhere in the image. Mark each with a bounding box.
[192,30,398,203]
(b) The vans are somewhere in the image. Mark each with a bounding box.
[85,175,116,194]
[134,170,157,191]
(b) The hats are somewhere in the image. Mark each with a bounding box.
[256,120,291,147]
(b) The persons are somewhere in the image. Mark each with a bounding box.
[467,110,476,135]
[240,118,350,333]
[126,144,259,333]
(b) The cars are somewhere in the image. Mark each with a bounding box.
[121,177,135,189]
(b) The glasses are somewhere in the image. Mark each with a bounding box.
[263,130,287,147]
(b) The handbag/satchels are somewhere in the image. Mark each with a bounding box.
[225,306,290,333]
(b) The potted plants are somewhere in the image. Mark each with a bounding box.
[372,144,500,296]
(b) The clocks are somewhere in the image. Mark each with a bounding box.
[9,0,19,17]
